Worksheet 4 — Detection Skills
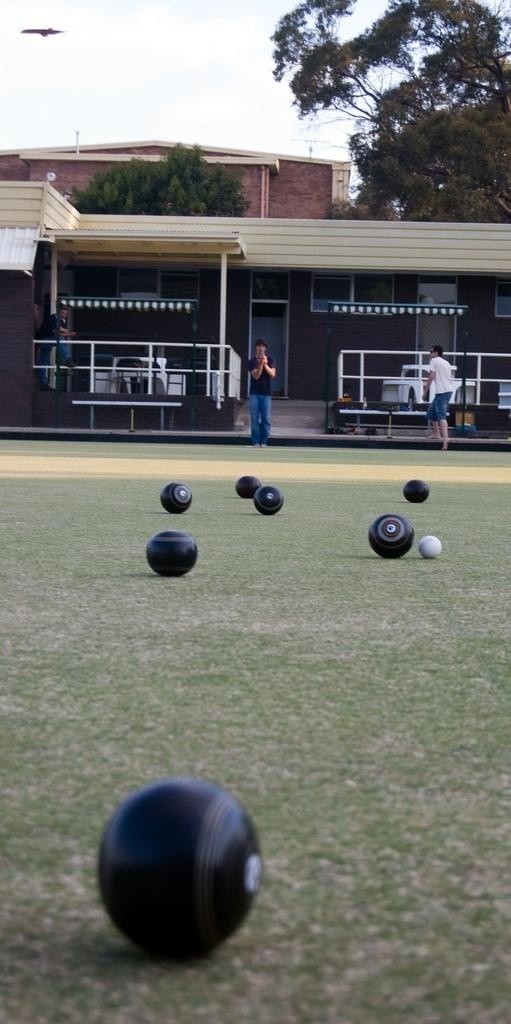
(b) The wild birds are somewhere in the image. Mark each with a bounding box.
[20,28,61,37]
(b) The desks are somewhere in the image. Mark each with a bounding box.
[399,363,457,403]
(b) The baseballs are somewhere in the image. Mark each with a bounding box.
[419,535,441,558]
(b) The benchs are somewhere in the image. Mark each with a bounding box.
[71,397,184,431]
[380,378,477,404]
[336,405,452,438]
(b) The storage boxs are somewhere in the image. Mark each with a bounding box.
[454,410,477,434]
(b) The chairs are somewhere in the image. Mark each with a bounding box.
[73,352,216,393]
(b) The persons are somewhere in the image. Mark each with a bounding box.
[246,338,277,448]
[35,303,78,392]
[422,344,453,450]
